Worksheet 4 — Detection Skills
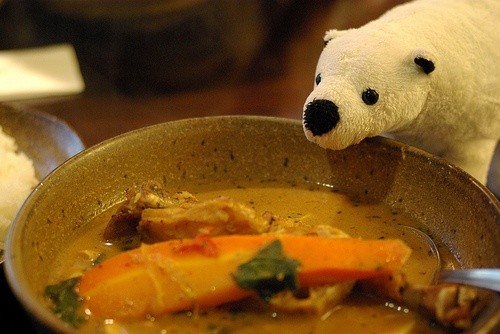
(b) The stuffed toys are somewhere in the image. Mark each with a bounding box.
[302,0,499,188]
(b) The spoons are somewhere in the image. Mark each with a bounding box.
[384,226,500,294]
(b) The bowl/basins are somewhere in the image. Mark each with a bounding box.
[6,115,499,334]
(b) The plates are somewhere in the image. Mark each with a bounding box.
[0,100,86,269]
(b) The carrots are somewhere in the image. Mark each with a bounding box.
[77,232,412,315]
